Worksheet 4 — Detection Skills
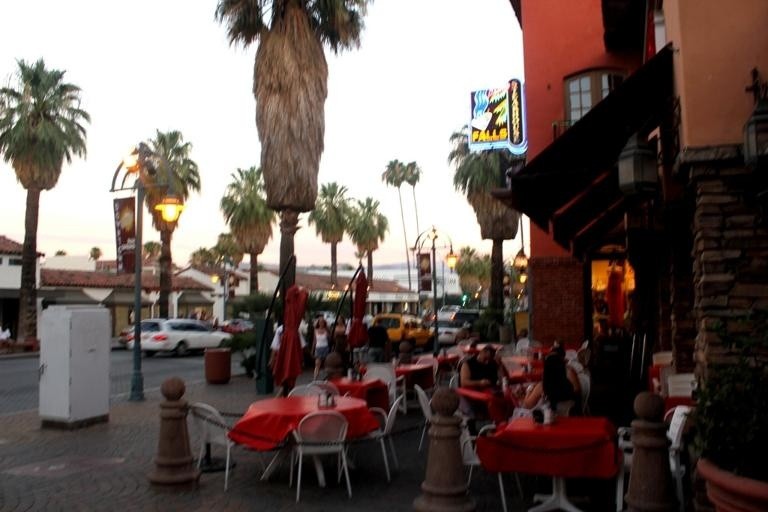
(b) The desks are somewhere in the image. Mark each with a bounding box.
[232,394,376,485]
[326,377,389,403]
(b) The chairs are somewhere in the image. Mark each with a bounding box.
[291,410,353,504]
[307,381,340,396]
[391,338,692,511]
[289,384,323,398]
[361,393,405,482]
[192,402,263,493]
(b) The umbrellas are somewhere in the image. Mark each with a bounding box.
[272,283,309,397]
[346,271,370,348]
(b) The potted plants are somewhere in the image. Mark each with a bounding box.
[691,365,768,512]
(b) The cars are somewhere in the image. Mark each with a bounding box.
[314,311,374,334]
[371,313,435,353]
[433,311,485,345]
[222,319,255,333]
[120,318,230,357]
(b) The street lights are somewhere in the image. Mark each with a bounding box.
[109,143,188,401]
[211,254,228,320]
[410,226,458,355]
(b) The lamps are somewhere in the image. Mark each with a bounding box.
[617,129,660,196]
[743,66,768,173]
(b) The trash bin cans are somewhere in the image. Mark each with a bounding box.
[204,347,230,385]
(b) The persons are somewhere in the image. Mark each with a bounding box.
[271,323,306,391]
[459,346,509,391]
[524,350,583,417]
[313,315,388,382]
[516,328,529,354]
